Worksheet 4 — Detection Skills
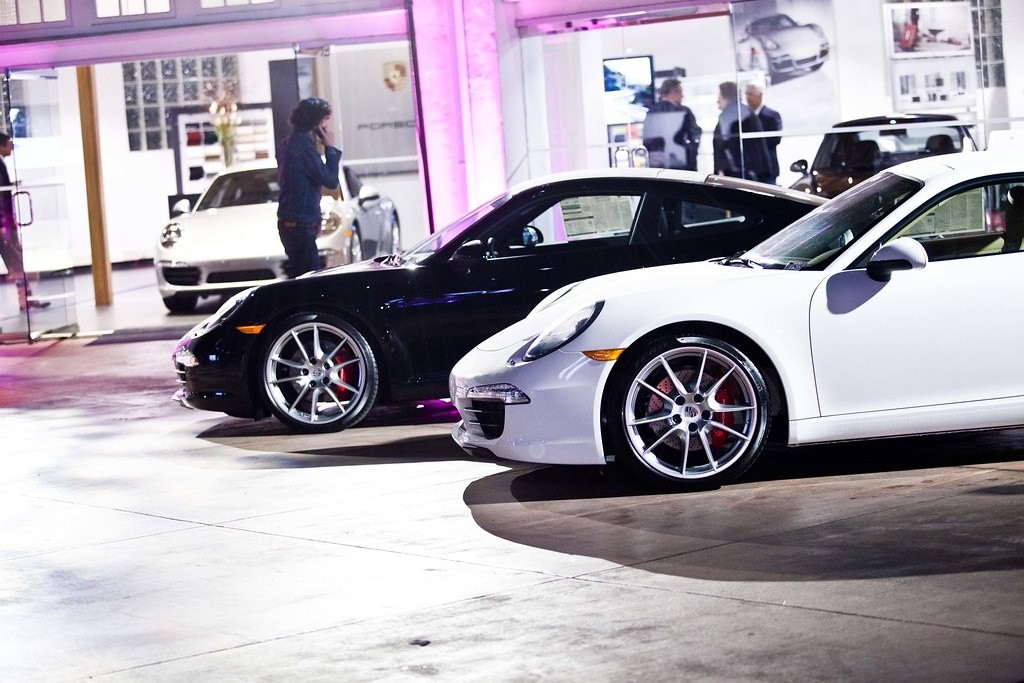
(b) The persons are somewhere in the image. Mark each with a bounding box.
[0,133,51,311]
[275,97,343,275]
[642,78,784,227]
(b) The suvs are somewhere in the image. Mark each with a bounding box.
[790,111,984,204]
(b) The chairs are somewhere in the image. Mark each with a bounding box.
[848,140,880,168]
[924,134,955,156]
[975,186,1024,254]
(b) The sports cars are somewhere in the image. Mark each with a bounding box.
[152,169,401,312]
[448,147,1024,492]
[737,15,833,83]
[172,165,853,433]
